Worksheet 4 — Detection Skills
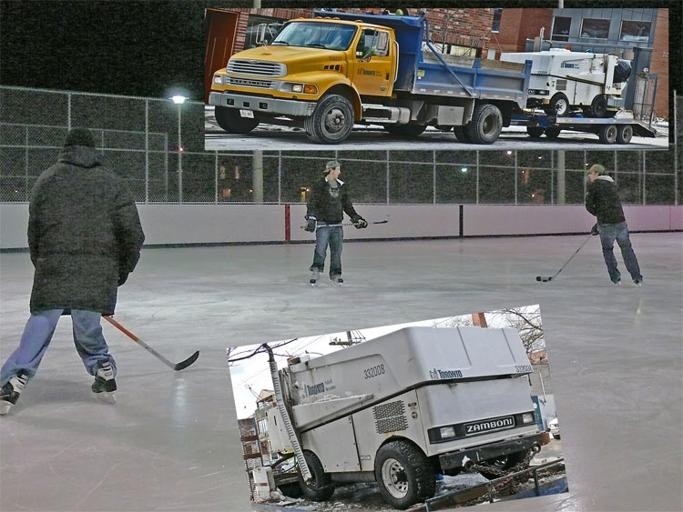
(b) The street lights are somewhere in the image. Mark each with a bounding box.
[638,66,651,121]
[165,92,190,202]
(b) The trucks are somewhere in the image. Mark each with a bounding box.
[201,9,535,144]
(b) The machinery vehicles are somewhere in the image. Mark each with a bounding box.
[258,319,549,506]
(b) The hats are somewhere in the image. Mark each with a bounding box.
[64,127,96,151]
[586,163,605,176]
[321,159,342,174]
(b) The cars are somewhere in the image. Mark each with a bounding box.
[548,415,559,441]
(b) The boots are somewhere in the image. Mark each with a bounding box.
[0,368,31,407]
[91,359,119,394]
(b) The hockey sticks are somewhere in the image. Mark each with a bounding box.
[535,232,594,282]
[101,314,199,371]
[300,219,389,229]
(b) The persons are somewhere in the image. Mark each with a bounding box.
[304,160,368,284]
[583,164,642,283]
[1,127,145,408]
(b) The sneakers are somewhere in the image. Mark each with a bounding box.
[329,270,343,283]
[309,267,321,283]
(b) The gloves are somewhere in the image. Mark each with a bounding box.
[304,217,318,233]
[352,217,368,230]
[591,223,600,236]
